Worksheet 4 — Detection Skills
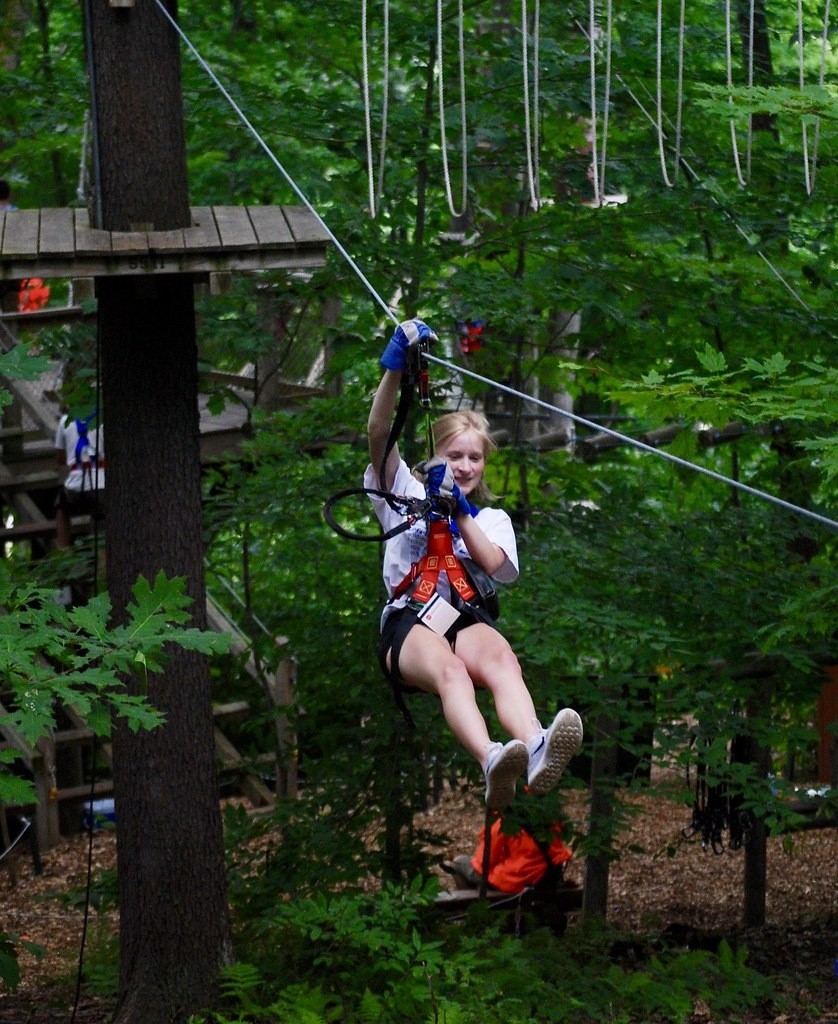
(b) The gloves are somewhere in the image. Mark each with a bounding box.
[424,457,471,516]
[380,319,440,372]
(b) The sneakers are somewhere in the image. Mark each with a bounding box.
[527,708,583,796]
[484,739,530,809]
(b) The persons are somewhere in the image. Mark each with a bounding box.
[54,414,106,551]
[364,319,582,810]
[451,786,572,893]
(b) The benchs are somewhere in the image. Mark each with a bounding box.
[397,879,584,937]
